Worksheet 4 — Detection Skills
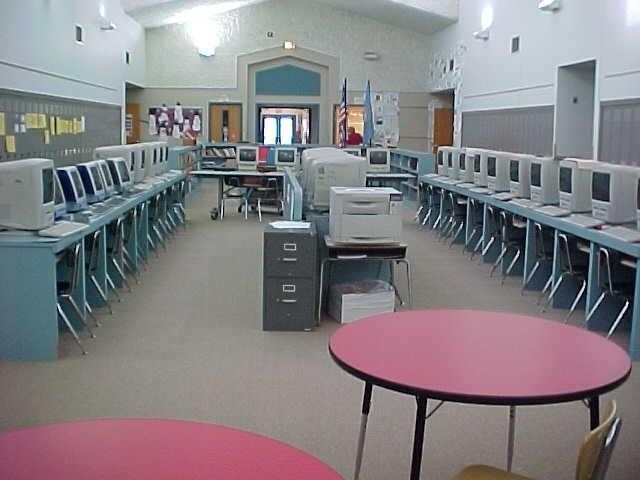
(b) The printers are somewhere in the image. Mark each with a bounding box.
[329,186,404,245]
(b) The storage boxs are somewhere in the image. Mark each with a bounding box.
[262,220,317,333]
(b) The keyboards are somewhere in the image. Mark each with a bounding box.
[424,174,439,178]
[104,198,126,207]
[435,176,452,183]
[94,206,113,215]
[492,192,519,201]
[469,188,495,194]
[602,225,640,243]
[37,220,88,238]
[124,189,143,198]
[442,180,463,186]
[456,183,476,189]
[135,170,182,189]
[509,199,543,209]
[561,215,605,228]
[536,206,571,217]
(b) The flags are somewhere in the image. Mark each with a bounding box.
[363,81,375,147]
[337,79,346,147]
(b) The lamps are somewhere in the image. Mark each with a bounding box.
[198,43,215,58]
[98,2,116,31]
[472,4,495,42]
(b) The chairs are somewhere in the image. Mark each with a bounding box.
[170,184,187,229]
[147,198,168,258]
[477,205,499,264]
[422,185,432,226]
[413,182,428,222]
[106,216,132,303]
[179,181,192,225]
[136,202,152,272]
[431,186,442,230]
[254,176,285,223]
[124,207,143,286]
[580,247,636,340]
[85,230,114,327]
[160,189,178,235]
[221,175,254,221]
[490,211,527,287]
[462,199,485,259]
[56,240,96,356]
[448,193,467,249]
[540,233,589,325]
[437,191,452,236]
[521,222,554,306]
[449,398,624,480]
[154,194,172,244]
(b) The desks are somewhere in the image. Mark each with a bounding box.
[418,173,639,362]
[328,308,633,480]
[0,169,188,361]
[190,169,285,219]
[0,419,346,480]
[365,172,416,187]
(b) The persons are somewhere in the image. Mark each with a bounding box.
[346,126,363,144]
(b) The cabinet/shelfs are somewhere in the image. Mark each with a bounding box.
[202,144,238,169]
[165,146,203,170]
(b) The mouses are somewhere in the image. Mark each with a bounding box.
[80,211,93,217]
[128,188,136,193]
[596,224,611,230]
[488,191,496,195]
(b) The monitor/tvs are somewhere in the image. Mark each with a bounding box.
[474,149,488,187]
[76,164,106,203]
[57,165,88,212]
[301,147,365,209]
[487,151,509,191]
[559,159,593,212]
[458,148,474,182]
[236,147,259,170]
[447,147,457,178]
[529,157,561,204]
[84,160,114,198]
[437,146,447,174]
[54,170,66,218]
[508,157,530,197]
[0,158,55,230]
[93,142,169,183]
[590,165,638,222]
[105,157,132,194]
[275,147,296,166]
[367,148,390,171]
[341,148,362,157]
